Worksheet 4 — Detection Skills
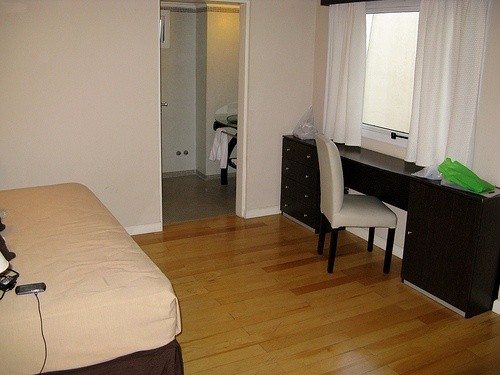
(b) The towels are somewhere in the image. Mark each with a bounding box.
[208,128,228,168]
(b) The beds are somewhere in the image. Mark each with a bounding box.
[0,183,183,375]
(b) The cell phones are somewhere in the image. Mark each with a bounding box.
[15,283,46,295]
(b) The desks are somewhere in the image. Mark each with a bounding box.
[213,116,238,186]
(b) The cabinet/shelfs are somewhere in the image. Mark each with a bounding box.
[280,135,500,319]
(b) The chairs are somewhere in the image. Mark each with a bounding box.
[315,132,397,274]
